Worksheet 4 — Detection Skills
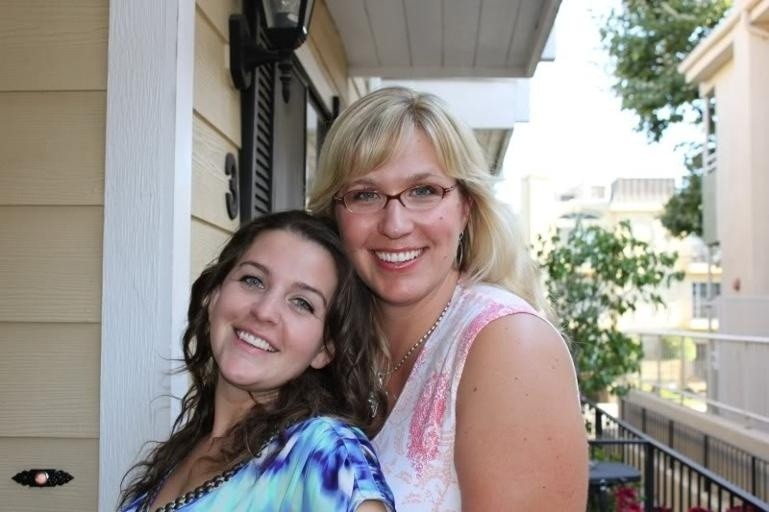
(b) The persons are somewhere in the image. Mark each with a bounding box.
[308,85,590,510]
[118,211,395,511]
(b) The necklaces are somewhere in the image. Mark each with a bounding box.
[140,437,278,510]
[369,297,451,417]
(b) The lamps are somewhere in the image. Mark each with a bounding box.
[228,1,314,105]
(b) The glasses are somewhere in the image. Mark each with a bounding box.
[327,182,459,216]
